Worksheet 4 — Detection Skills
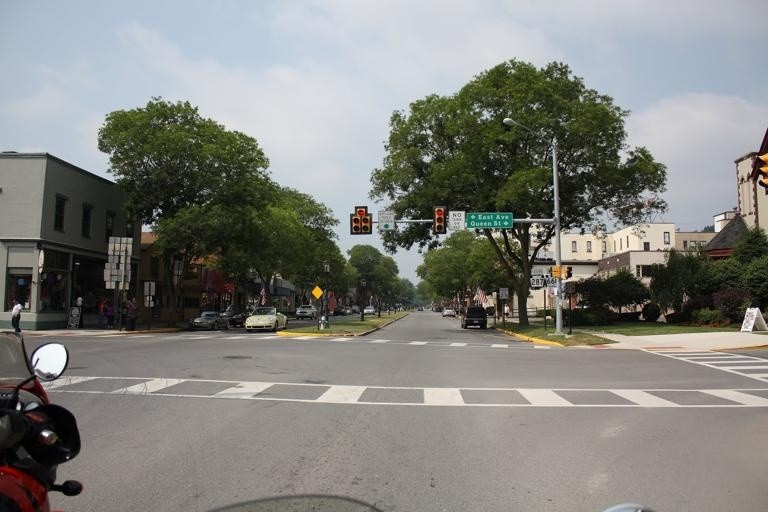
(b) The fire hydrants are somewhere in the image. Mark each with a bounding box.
[318,315,329,330]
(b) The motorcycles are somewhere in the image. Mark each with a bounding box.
[0,331,84,511]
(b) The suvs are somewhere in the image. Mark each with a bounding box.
[296,305,318,320]
[221,303,256,327]
[461,306,487,329]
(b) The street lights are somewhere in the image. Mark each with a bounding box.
[377,285,384,317]
[319,258,332,328]
[356,276,369,320]
[394,293,397,313]
[502,116,563,336]
[386,289,393,315]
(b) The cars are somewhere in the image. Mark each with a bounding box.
[441,307,456,317]
[364,306,375,315]
[332,306,352,316]
[417,301,441,312]
[186,311,229,331]
[375,303,409,312]
[351,306,360,315]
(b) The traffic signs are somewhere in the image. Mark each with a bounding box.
[448,209,464,231]
[376,209,395,232]
[466,212,513,227]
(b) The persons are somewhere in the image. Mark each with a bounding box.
[503,304,510,321]
[127,297,136,318]
[100,295,107,328]
[105,300,114,330]
[12,299,24,332]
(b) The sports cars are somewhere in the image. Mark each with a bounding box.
[245,307,288,332]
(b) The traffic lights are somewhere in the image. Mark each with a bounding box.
[435,207,444,233]
[549,265,559,279]
[351,216,360,232]
[362,216,370,232]
[756,152,767,194]
[356,208,365,217]
[561,265,573,281]
